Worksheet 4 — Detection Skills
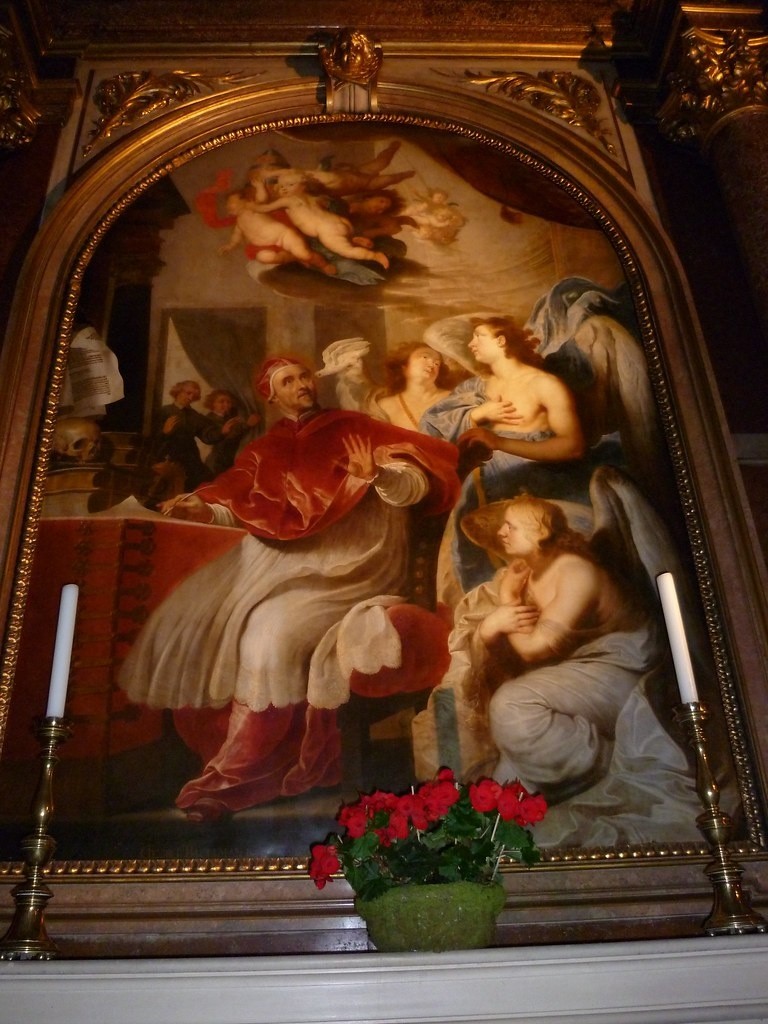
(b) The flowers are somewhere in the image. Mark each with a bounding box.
[308,771,549,896]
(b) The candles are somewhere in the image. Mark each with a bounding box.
[45,583,81,717]
[655,572,699,707]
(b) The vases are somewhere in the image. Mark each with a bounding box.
[355,883,508,953]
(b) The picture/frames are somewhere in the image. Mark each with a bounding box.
[0,34,768,881]
